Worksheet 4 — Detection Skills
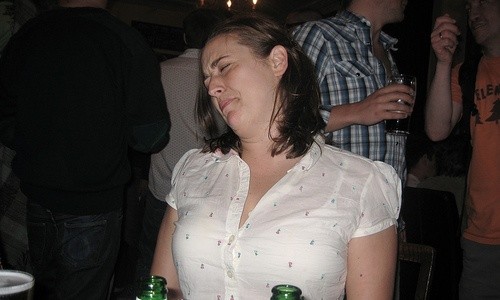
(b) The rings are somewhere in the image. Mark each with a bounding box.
[439,32,443,39]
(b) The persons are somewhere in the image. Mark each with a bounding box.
[150,10,403,299]
[1,1,499,300]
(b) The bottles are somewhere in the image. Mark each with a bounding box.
[270,285,302,300]
[136,276,168,300]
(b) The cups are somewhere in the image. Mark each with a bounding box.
[386,74,417,135]
[0,270,35,300]
